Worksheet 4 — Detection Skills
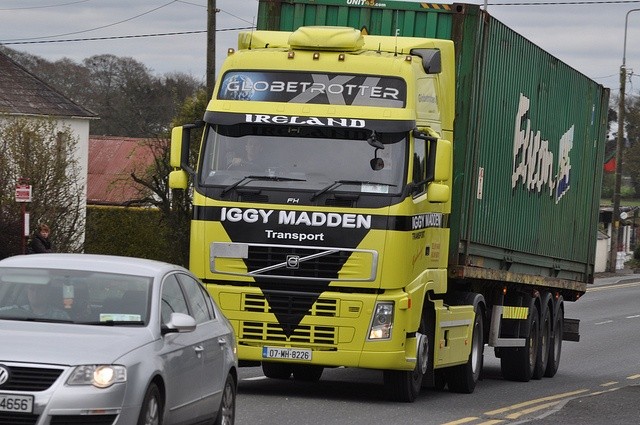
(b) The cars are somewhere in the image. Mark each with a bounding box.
[1,252,239,425]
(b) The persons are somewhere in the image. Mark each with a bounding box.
[227,135,267,169]
[31,224,53,251]
[1,284,71,321]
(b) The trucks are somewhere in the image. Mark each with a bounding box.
[169,0,611,404]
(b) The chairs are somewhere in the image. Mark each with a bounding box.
[117,286,148,315]
